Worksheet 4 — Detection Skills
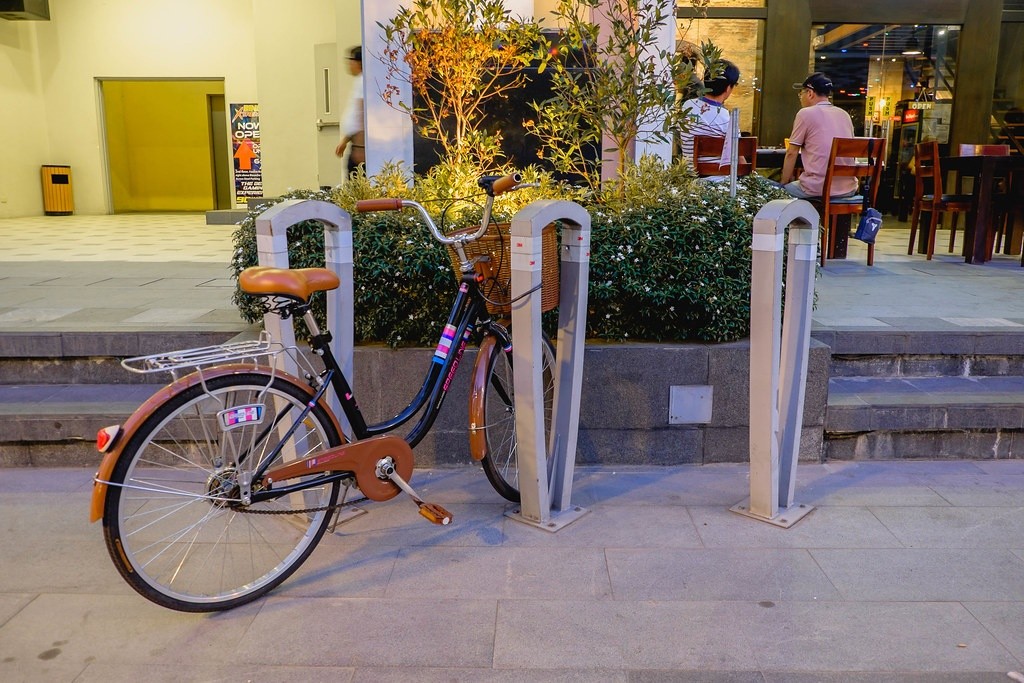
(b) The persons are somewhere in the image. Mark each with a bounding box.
[334,45,365,178]
[676,52,705,165]
[780,72,860,200]
[679,59,786,214]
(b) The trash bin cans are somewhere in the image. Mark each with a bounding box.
[41,165,75,216]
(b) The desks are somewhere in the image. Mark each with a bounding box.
[921,155,1024,264]
[756,149,807,169]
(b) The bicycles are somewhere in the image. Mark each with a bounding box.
[91,173,558,613]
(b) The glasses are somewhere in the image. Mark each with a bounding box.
[797,90,809,98]
[734,82,738,86]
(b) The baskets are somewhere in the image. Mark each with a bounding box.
[445,222,559,314]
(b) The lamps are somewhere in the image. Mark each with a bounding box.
[901,26,924,55]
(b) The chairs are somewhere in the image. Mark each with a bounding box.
[694,134,756,180]
[908,142,974,261]
[812,137,887,266]
[949,143,1006,253]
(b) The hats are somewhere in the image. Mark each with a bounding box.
[793,72,833,93]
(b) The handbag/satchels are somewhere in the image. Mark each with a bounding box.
[855,208,882,244]
